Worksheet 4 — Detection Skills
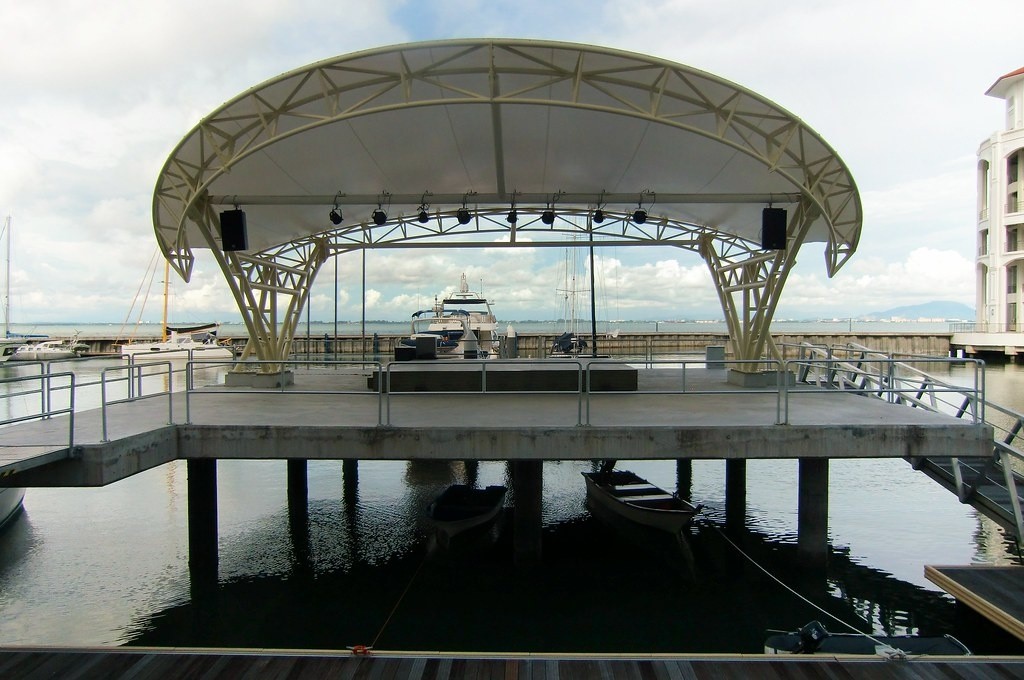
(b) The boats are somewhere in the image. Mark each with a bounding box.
[424,483,508,554]
[761,627,975,655]
[120,331,234,363]
[578,467,705,534]
[408,272,500,360]
[7,340,75,360]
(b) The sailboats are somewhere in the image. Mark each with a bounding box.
[110,243,232,350]
[548,214,613,359]
